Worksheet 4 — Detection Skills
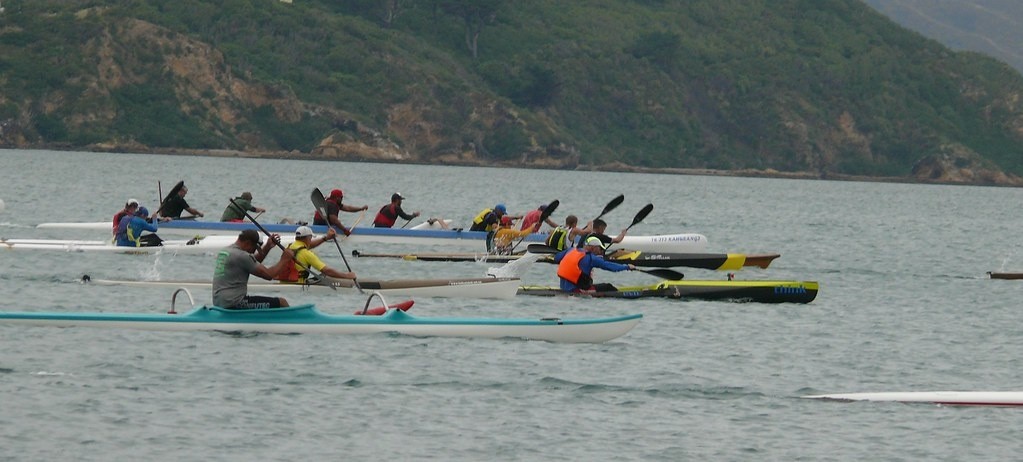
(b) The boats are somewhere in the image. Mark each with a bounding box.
[37,217,711,258]
[515,277,820,305]
[800,387,1023,407]
[986,270,1023,281]
[0,230,324,256]
[0,304,645,344]
[349,244,782,269]
[82,274,521,300]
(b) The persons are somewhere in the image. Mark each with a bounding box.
[519,204,558,234]
[469,204,524,232]
[219,192,266,223]
[116,206,165,247]
[312,188,368,236]
[212,229,295,309]
[576,218,627,257]
[112,197,138,245]
[280,225,357,285]
[158,184,204,220]
[486,216,538,255]
[555,237,636,291]
[370,192,420,229]
[545,215,594,251]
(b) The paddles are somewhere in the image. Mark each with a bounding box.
[254,211,264,220]
[310,187,363,293]
[602,202,654,251]
[511,219,521,230]
[341,210,366,241]
[147,181,185,223]
[509,199,561,251]
[228,196,339,292]
[582,193,624,230]
[401,218,412,229]
[180,215,203,219]
[527,242,685,280]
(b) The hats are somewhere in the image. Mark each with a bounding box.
[126,199,138,207]
[392,193,405,199]
[135,207,148,216]
[239,230,263,246]
[585,237,606,250]
[331,190,343,197]
[540,204,551,216]
[496,204,507,214]
[501,215,514,225]
[296,226,314,237]
